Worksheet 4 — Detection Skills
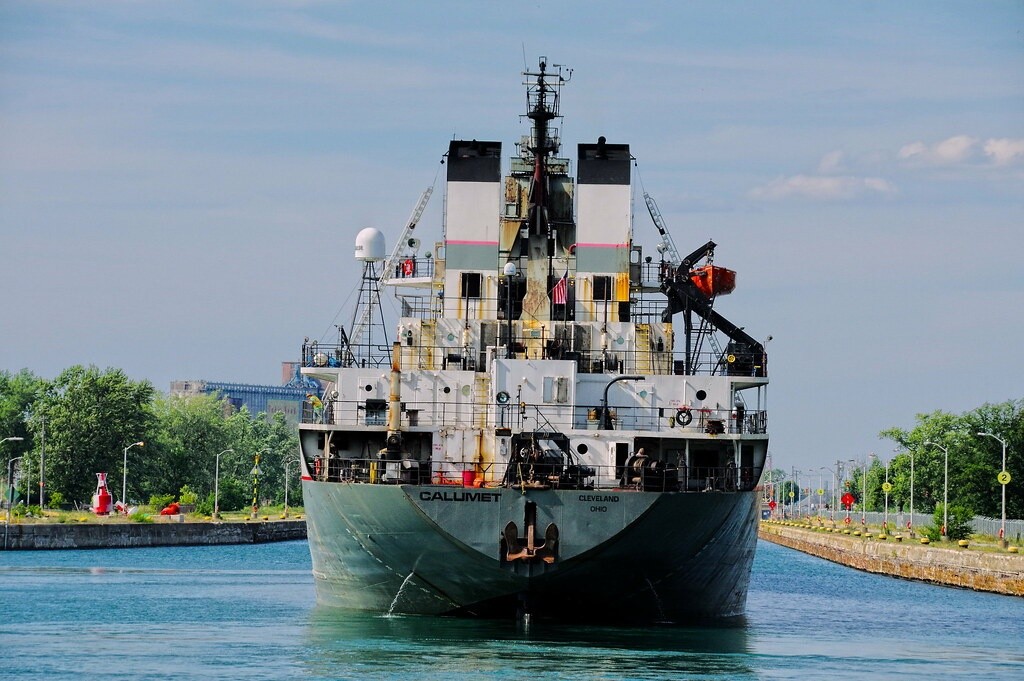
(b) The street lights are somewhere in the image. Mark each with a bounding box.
[977,432,1007,541]
[923,441,948,535]
[892,448,914,534]
[6,456,25,522]
[781,453,888,536]
[213,448,234,520]
[247,447,272,519]
[120,441,144,515]
[282,459,300,518]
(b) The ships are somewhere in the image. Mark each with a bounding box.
[297,40,772,633]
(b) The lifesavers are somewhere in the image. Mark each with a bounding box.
[316,460,322,475]
[403,260,413,275]
[676,410,692,425]
